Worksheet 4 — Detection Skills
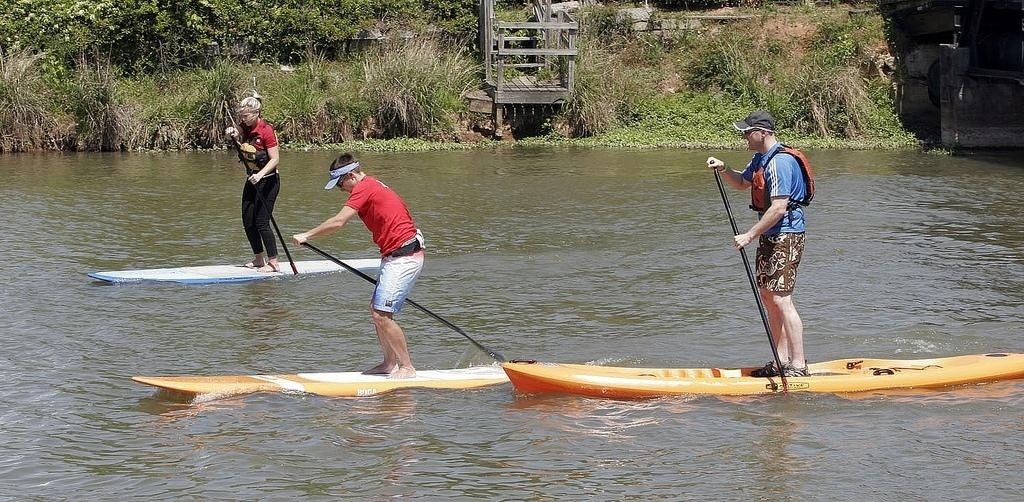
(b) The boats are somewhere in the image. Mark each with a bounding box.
[81,256,384,288]
[117,364,523,398]
[500,348,1024,397]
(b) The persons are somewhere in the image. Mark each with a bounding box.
[224,97,282,273]
[706,111,808,377]
[292,154,425,379]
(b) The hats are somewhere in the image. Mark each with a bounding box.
[323,160,361,192]
[731,110,777,135]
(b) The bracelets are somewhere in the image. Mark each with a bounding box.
[718,163,728,173]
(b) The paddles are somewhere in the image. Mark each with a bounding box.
[302,242,509,363]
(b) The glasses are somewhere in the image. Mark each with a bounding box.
[743,129,761,134]
[335,174,349,188]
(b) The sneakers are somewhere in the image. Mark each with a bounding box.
[779,360,810,377]
[750,357,791,377]
